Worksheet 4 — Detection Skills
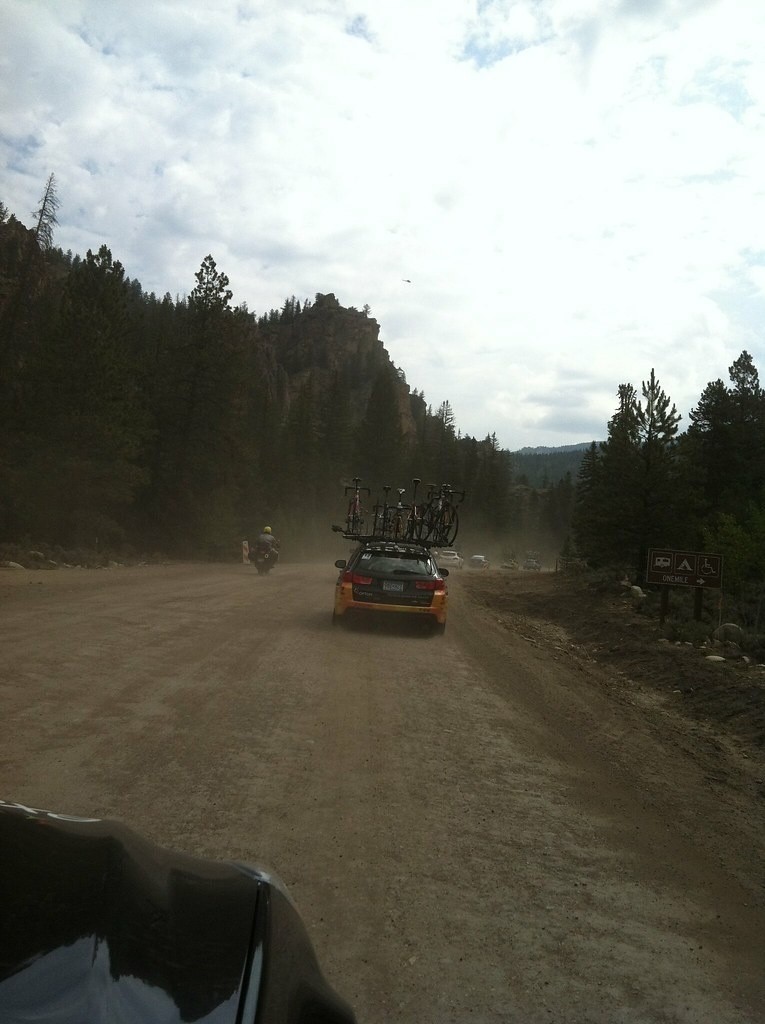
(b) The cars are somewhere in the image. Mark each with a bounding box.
[522,559,541,571]
[439,550,463,569]
[500,559,519,570]
[0,798,360,1024]
[469,555,489,567]
[333,537,449,635]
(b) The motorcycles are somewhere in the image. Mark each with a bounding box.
[249,539,280,576]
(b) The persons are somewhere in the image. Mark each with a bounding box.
[256,526,279,569]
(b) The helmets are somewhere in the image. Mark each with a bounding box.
[263,526,271,533]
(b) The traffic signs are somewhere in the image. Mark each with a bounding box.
[645,547,724,590]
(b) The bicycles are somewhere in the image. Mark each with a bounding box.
[330,474,466,547]
